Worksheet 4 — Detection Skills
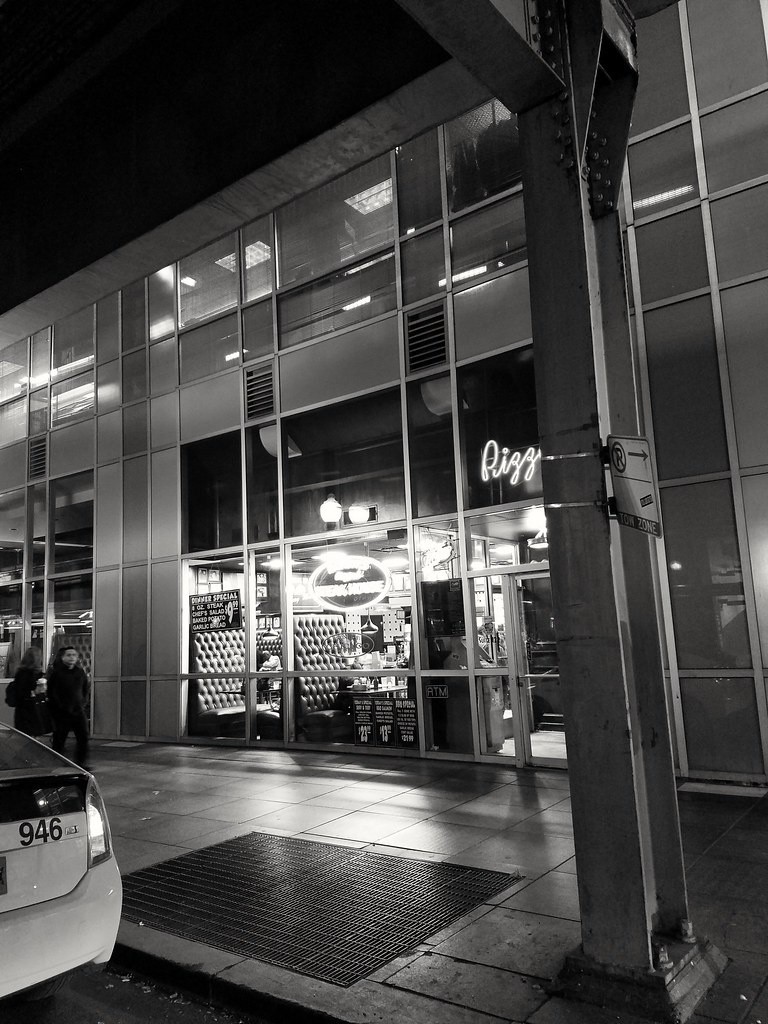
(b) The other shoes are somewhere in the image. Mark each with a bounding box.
[80,763,92,771]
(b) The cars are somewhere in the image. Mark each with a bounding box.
[0,721,125,1016]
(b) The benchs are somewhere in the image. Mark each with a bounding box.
[190,625,275,735]
[55,633,90,706]
[290,613,356,741]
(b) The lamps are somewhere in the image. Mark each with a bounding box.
[360,542,379,633]
[262,556,278,640]
[347,498,370,526]
[320,491,343,523]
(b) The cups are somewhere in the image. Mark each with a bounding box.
[37,678,47,693]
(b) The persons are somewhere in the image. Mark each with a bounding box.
[258,650,282,690]
[6,645,52,736]
[46,646,93,772]
[333,664,362,709]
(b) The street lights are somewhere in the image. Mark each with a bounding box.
[318,493,343,549]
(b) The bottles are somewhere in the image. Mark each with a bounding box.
[353,676,379,690]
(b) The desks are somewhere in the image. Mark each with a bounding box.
[219,689,281,713]
[330,685,407,699]
